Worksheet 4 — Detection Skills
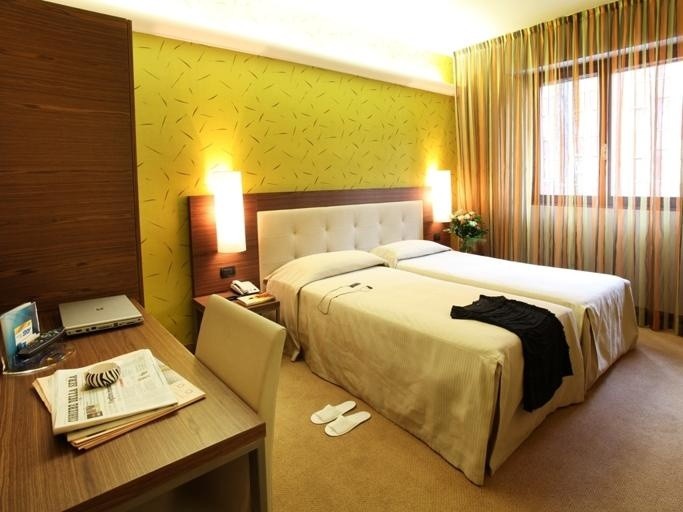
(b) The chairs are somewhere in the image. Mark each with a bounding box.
[186,292,288,512]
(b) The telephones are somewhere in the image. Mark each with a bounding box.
[230,280,260,295]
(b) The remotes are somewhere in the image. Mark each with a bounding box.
[17,325,65,356]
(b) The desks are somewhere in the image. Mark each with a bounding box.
[0,295,266,512]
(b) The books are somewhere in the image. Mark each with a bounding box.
[30,350,206,451]
[235,290,277,308]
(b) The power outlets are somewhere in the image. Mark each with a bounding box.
[221,268,236,278]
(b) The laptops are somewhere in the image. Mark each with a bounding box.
[58,294,144,336]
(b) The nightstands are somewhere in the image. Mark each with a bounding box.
[193,280,280,322]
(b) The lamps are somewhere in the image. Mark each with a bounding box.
[213,164,247,253]
[429,171,453,224]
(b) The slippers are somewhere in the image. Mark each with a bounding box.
[311,400,356,425]
[324,411,371,437]
